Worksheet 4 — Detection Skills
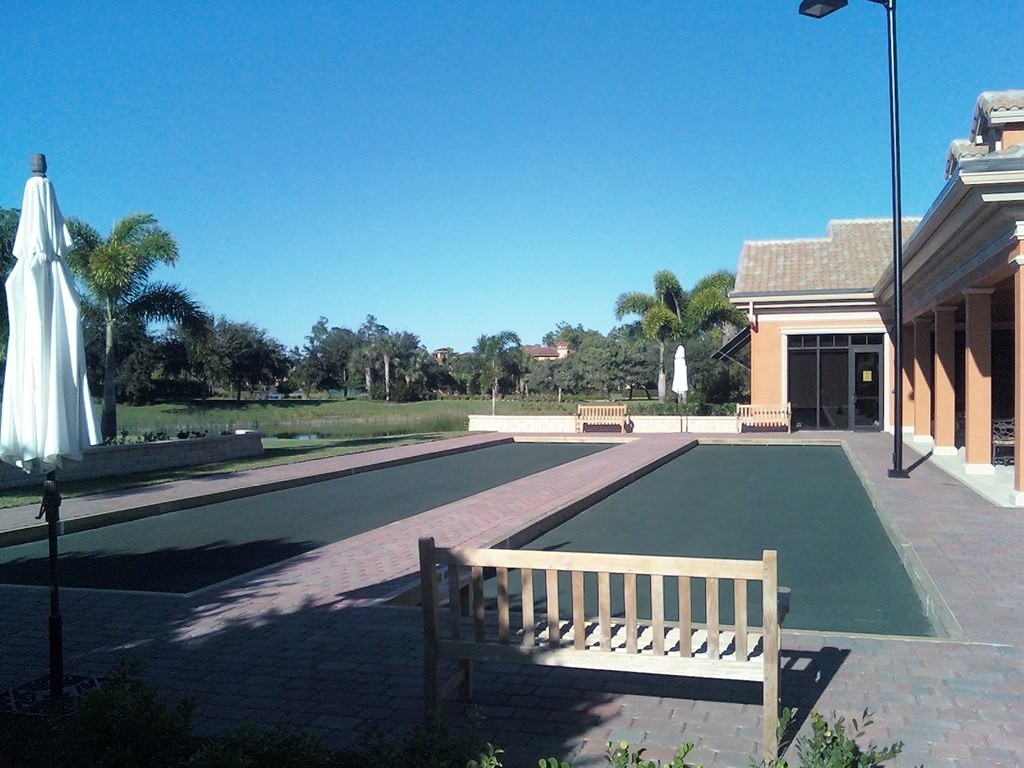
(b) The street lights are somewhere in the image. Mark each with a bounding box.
[799,0,909,479]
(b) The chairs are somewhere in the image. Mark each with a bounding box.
[992,417,1015,467]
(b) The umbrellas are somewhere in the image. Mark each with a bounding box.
[672,344,689,433]
[0,152,104,704]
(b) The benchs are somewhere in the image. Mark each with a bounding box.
[418,537,782,761]
[575,404,628,434]
[736,402,792,434]
